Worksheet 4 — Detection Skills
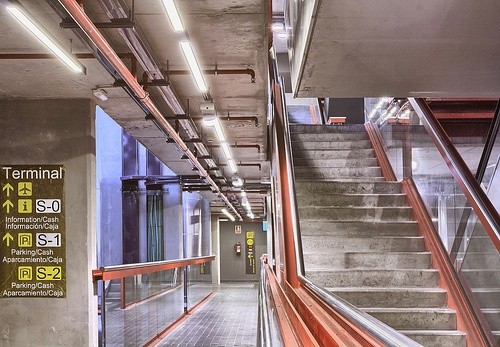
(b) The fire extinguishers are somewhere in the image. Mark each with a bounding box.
[236,242,241,256]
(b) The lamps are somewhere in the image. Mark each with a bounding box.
[159,0,256,221]
[6,3,86,79]
